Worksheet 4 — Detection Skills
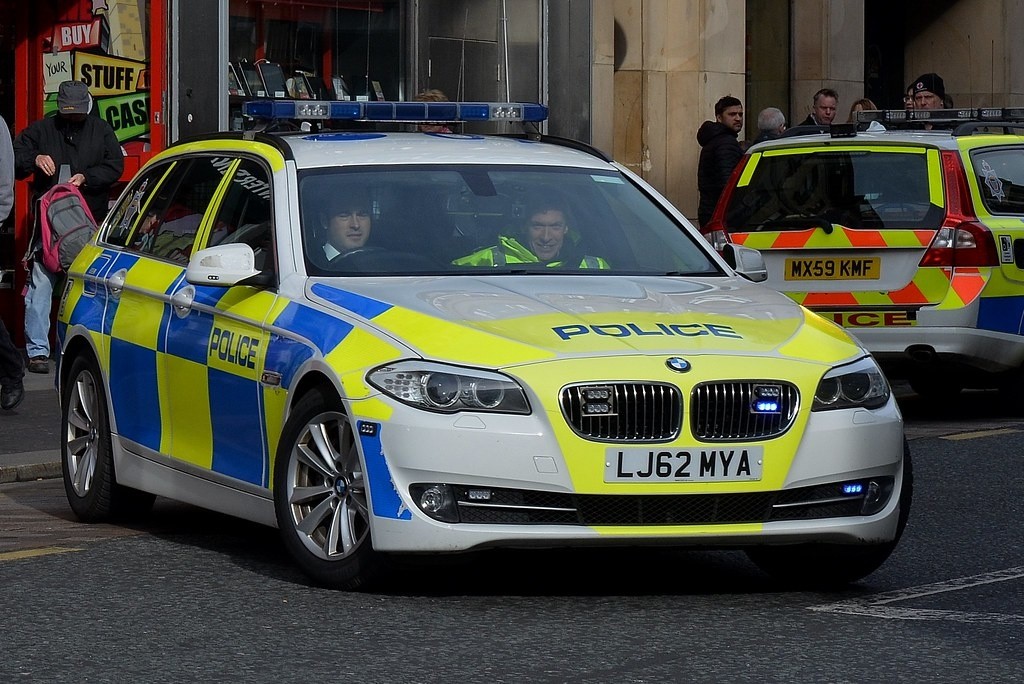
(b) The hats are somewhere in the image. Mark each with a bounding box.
[57,81,90,114]
[913,73,945,100]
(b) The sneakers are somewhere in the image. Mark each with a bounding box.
[29,356,49,374]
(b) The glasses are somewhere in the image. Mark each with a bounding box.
[904,96,913,102]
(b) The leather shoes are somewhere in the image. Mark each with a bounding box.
[0,364,25,412]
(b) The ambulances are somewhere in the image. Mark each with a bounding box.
[57,103,913,588]
[698,109,1024,422]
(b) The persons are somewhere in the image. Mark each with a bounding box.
[451,198,611,269]
[695,96,743,226]
[797,89,839,136]
[904,73,978,131]
[414,90,454,133]
[752,108,786,146]
[319,192,371,261]
[847,98,877,123]
[0,81,126,414]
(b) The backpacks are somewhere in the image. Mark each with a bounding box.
[22,164,99,296]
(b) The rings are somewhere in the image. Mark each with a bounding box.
[44,164,47,167]
